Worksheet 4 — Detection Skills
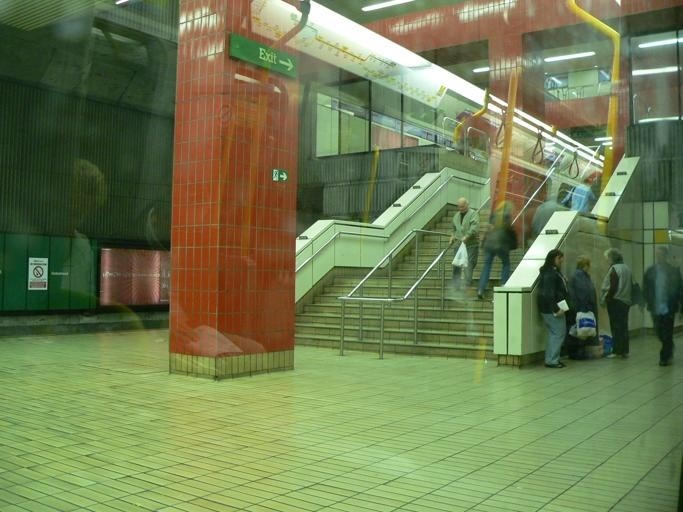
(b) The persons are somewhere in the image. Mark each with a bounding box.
[0,157,110,311]
[449,178,597,299]
[539,246,683,368]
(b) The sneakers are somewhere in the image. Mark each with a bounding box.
[544,353,628,369]
[477,292,485,299]
[657,353,671,365]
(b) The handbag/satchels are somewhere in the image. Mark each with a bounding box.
[551,299,569,316]
[626,269,642,305]
[506,228,518,250]
